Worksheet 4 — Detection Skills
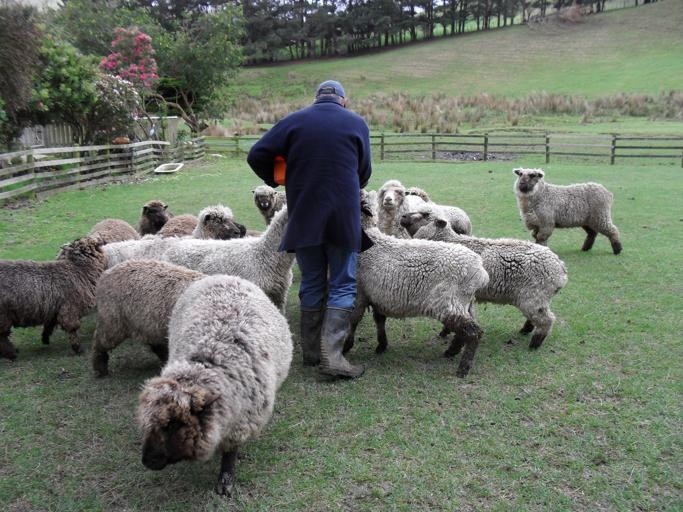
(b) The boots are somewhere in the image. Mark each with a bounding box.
[320,307,364,378]
[300,311,323,367]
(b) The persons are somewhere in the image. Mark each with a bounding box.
[246,78,372,380]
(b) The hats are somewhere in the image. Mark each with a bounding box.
[316,80,345,99]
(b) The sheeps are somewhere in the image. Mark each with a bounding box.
[250,183,288,228]
[512,166,623,256]
[137,273,295,498]
[0,233,106,361]
[411,218,569,350]
[91,258,210,380]
[360,178,471,238]
[343,200,490,379]
[84,198,298,310]
